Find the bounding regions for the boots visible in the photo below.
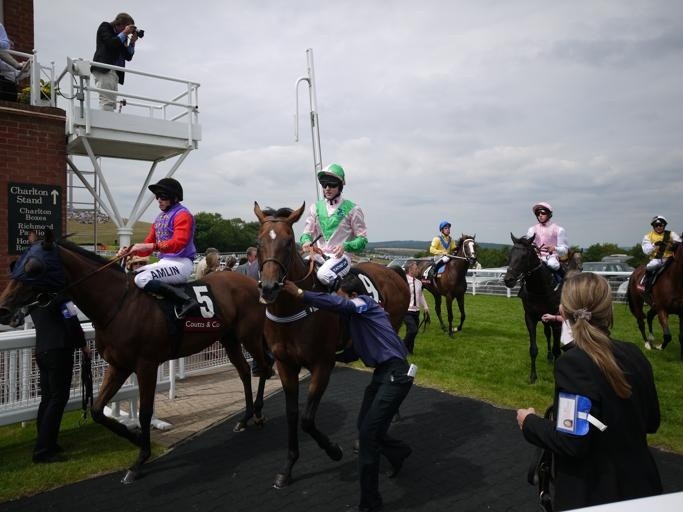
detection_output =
[144,280,199,321]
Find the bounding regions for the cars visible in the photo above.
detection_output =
[582,254,637,302]
[357,257,372,265]
[386,259,434,272]
[466,267,522,296]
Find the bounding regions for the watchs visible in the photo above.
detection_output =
[295,288,303,299]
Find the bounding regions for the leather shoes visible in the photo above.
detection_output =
[386,445,412,477]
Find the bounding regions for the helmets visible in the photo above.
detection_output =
[317,164,345,186]
[148,178,183,202]
[439,220,451,231]
[534,209,548,215]
[650,215,668,226]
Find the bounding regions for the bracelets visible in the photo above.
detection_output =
[555,316,557,322]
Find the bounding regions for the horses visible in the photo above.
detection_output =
[253,200,411,491]
[0,227,269,485]
[416,233,478,339]
[504,232,566,385]
[564,248,584,273]
[626,240,683,360]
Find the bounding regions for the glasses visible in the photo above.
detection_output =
[156,191,169,201]
[320,179,339,189]
[531,201,552,213]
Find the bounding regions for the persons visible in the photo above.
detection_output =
[29,299,92,465]
[90,13,138,111]
[0,21,30,102]
[116,177,200,318]
[301,163,368,292]
[526,202,569,279]
[637,215,682,291]
[403,260,430,354]
[516,272,664,511]
[282,273,414,511]
[126,246,261,284]
[427,221,457,285]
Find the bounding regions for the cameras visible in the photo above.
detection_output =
[133,28,144,39]
